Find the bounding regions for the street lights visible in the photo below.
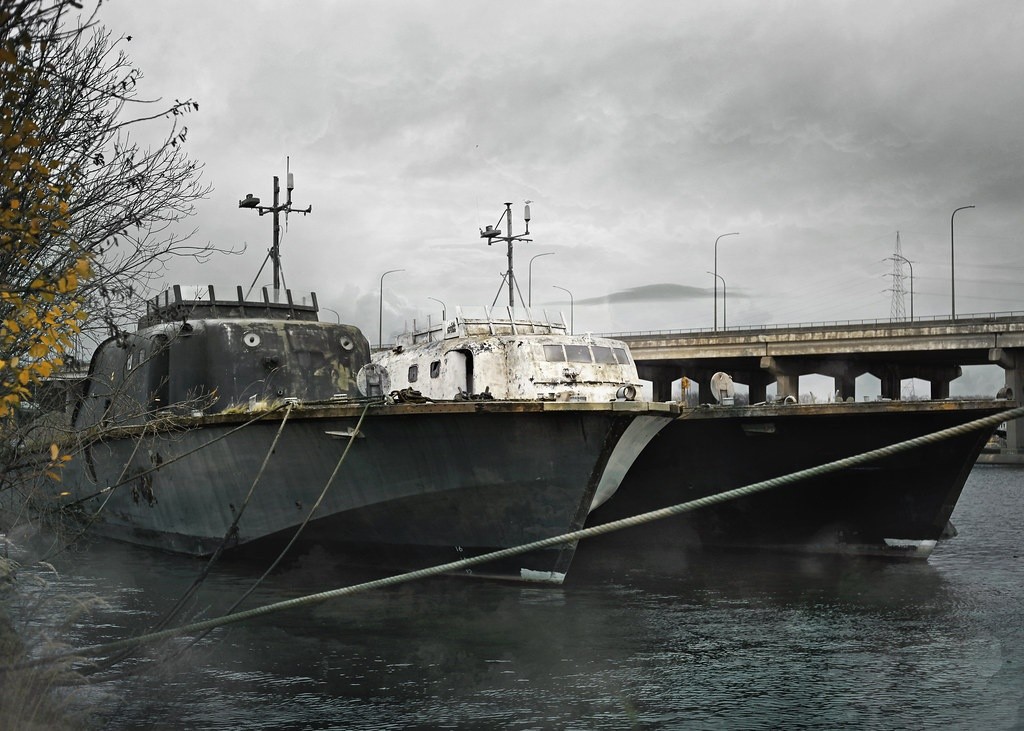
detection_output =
[707,272,726,331]
[428,297,446,321]
[553,286,573,335]
[893,254,913,322]
[715,233,739,331]
[951,205,975,320]
[380,269,406,349]
[529,253,555,307]
[322,308,339,324]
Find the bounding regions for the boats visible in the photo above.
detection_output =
[370,200,1018,562]
[0,156,684,584]
